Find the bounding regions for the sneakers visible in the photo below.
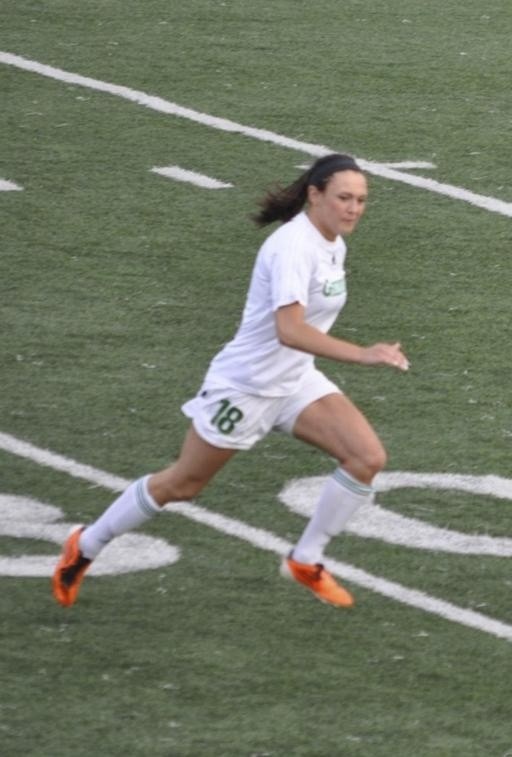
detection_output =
[53,524,94,609]
[279,547,356,609]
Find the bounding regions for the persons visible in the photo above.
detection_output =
[50,154,410,608]
